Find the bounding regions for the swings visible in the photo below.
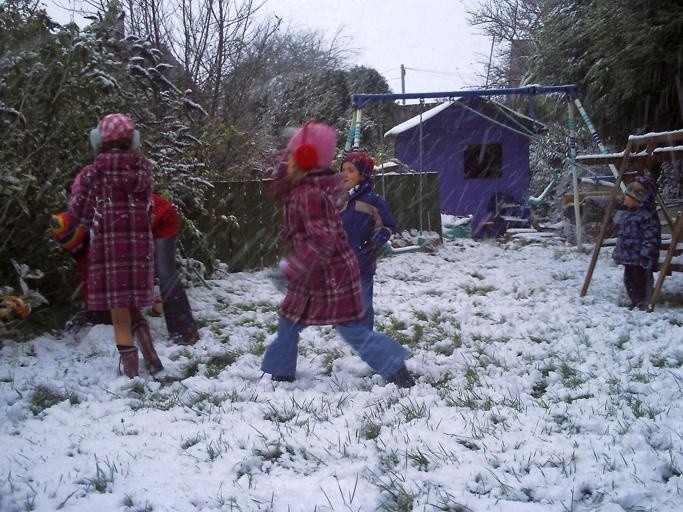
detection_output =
[484,94,536,225]
[453,95,616,188]
[378,98,425,254]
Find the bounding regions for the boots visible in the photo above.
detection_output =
[116,345,139,379]
[133,320,163,372]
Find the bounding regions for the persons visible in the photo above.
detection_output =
[261,122,415,387]
[58,113,163,378]
[50,188,199,345]
[612,177,659,308]
[335,153,395,329]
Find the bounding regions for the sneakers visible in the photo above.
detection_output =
[177,331,199,346]
[394,366,412,387]
[271,377,296,382]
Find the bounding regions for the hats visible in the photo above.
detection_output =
[288,125,337,169]
[624,181,647,203]
[97,114,135,149]
[51,213,88,255]
[340,150,374,181]
